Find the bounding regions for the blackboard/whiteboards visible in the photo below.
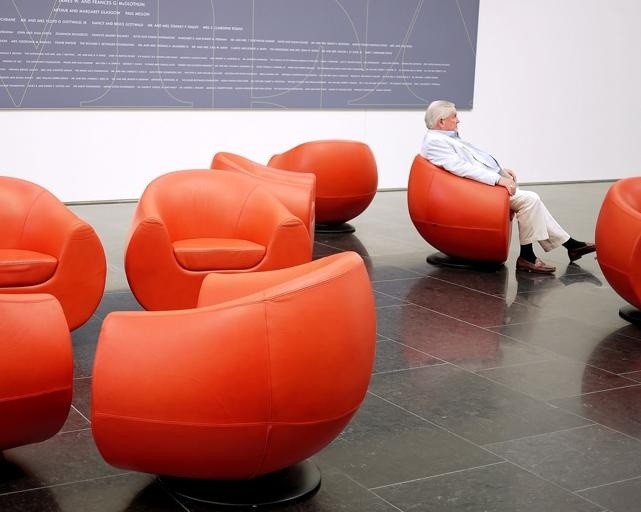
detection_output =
[0,0,480,111]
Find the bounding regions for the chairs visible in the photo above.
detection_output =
[594,177,641,328]
[270,142,378,236]
[210,152,317,252]
[0,176,108,331]
[91,253,377,512]
[125,170,316,311]
[408,154,513,271]
[0,293,73,452]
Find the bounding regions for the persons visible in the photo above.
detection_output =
[476,259,604,372]
[418,98,597,274]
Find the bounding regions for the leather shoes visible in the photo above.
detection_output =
[568,240,596,261]
[516,254,556,274]
